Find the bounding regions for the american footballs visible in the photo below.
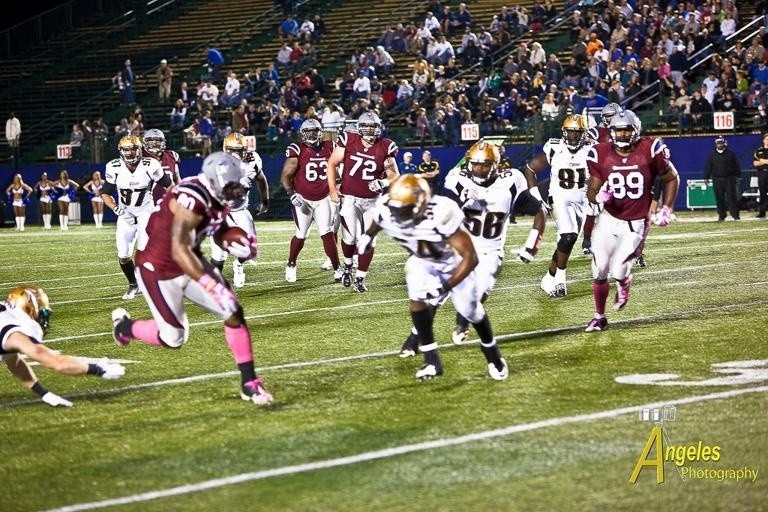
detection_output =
[214,227,248,251]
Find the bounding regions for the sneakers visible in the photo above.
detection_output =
[321,258,369,294]
[122,285,139,301]
[510,247,536,262]
[540,246,645,331]
[285,260,297,282]
[240,376,274,407]
[399,325,509,381]
[111,307,131,347]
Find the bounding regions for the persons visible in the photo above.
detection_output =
[110,151,274,408]
[398,141,548,361]
[356,170,510,381]
[0,0,767,300]
[585,111,680,334]
[1,284,127,407]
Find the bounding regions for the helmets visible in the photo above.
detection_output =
[561,103,642,152]
[117,128,166,165]
[465,142,501,185]
[383,174,431,229]
[343,111,383,145]
[201,132,256,212]
[300,119,323,150]
[5,285,52,336]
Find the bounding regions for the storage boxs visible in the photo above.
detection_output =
[686,178,719,210]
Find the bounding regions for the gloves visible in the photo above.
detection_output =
[329,201,341,233]
[85,357,126,380]
[197,273,239,313]
[368,178,390,192]
[222,234,258,263]
[650,205,673,227]
[457,188,478,209]
[286,187,305,207]
[352,235,373,269]
[29,379,74,407]
[528,185,552,217]
[418,282,455,308]
[594,180,615,212]
[111,204,127,218]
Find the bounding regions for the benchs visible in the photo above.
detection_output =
[742,176,767,205]
[1,0,768,161]
